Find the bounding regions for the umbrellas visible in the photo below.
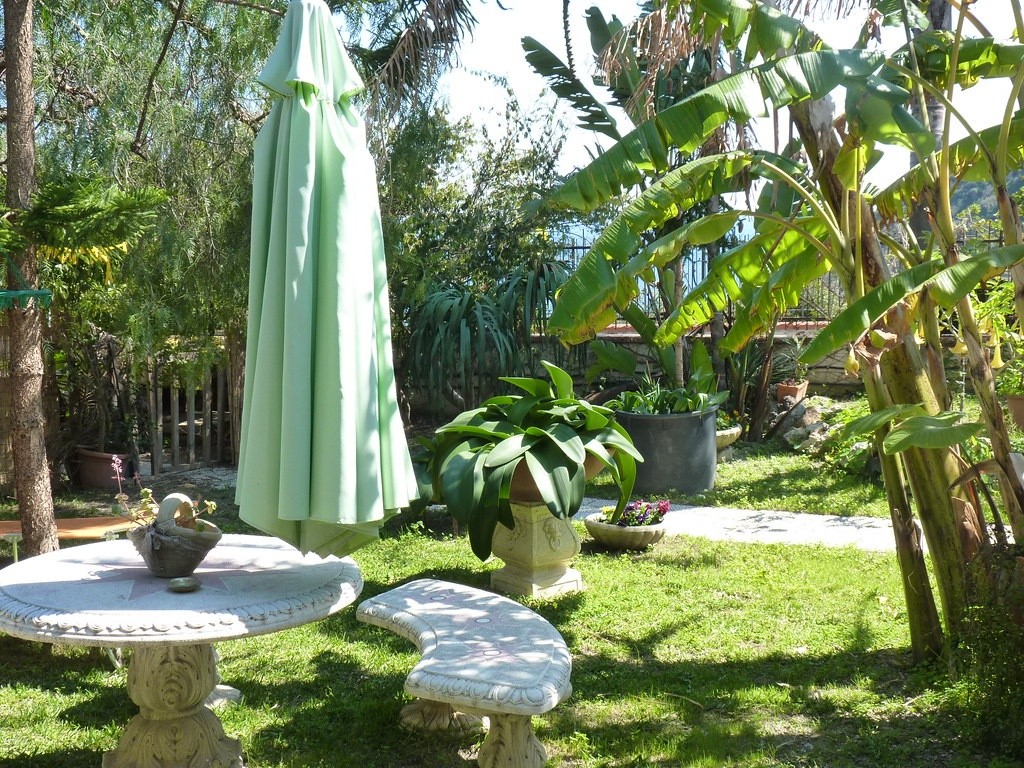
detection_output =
[235,0,425,559]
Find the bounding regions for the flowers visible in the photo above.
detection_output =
[600,489,680,527]
[102,455,218,541]
[716,408,741,431]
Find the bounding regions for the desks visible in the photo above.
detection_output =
[2,533,364,767]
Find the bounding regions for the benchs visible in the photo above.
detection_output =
[355,579,573,767]
[0,515,157,564]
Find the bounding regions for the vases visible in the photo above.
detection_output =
[585,511,666,548]
[716,423,742,450]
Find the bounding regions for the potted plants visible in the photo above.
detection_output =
[601,363,729,495]
[408,359,648,564]
[996,360,1024,432]
[776,334,809,403]
[58,324,151,489]
